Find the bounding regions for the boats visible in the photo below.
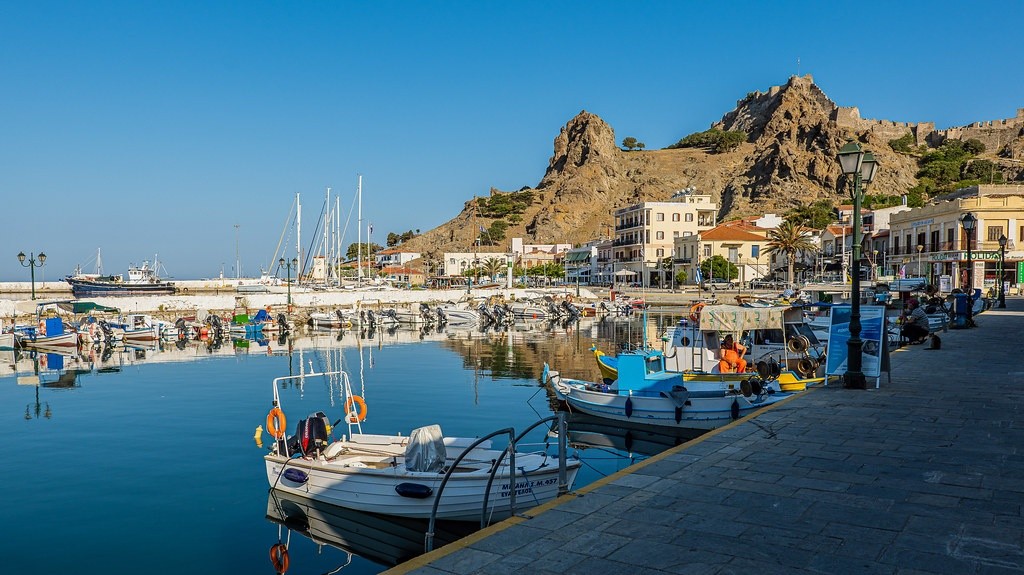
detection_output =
[63,247,178,297]
[541,359,796,430]
[702,278,735,291]
[748,276,797,290]
[544,407,712,458]
[267,369,581,525]
[1,286,646,371]
[661,284,989,378]
[266,488,484,568]
[590,310,840,397]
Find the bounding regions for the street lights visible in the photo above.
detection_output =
[17,250,46,300]
[873,250,879,265]
[233,224,242,280]
[917,245,924,279]
[997,234,1008,308]
[756,256,760,278]
[960,212,979,329]
[834,137,882,391]
[738,253,743,285]
[572,260,583,296]
[461,260,476,300]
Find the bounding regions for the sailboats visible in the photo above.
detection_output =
[255,174,374,291]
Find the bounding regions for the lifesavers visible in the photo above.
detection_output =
[343,394,368,423]
[266,408,287,438]
[688,302,708,321]
[270,543,289,573]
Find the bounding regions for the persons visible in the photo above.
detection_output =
[901,299,930,344]
[720,334,747,373]
